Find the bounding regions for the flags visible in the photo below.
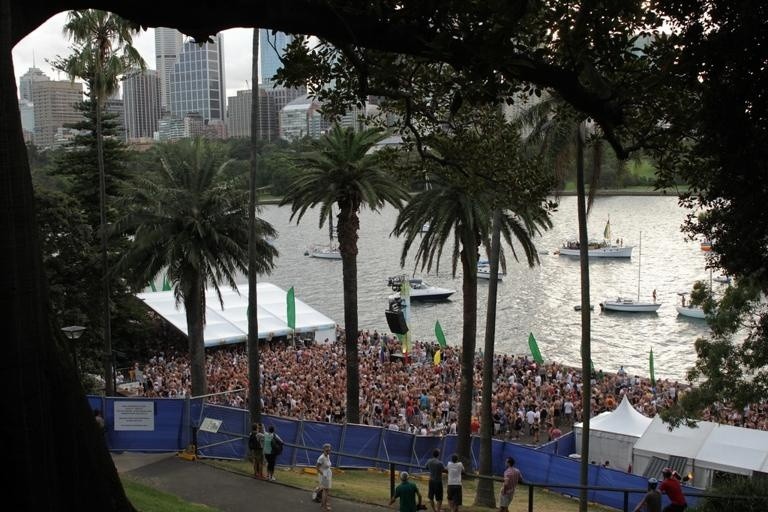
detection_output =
[604,221,611,239]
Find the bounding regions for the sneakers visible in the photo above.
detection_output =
[254,472,276,482]
[319,502,332,512]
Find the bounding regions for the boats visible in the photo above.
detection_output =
[557,212,638,261]
[381,272,456,304]
[474,258,504,282]
[418,221,431,233]
[303,226,341,263]
[712,276,730,283]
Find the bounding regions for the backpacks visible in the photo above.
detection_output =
[248,432,260,450]
[271,433,284,455]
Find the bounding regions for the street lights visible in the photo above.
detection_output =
[59,323,88,381]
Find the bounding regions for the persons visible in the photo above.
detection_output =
[633,478,661,512]
[447,455,465,512]
[498,457,522,512]
[656,468,687,512]
[563,237,622,249]
[389,472,422,512]
[425,450,448,512]
[653,289,656,302]
[317,444,333,511]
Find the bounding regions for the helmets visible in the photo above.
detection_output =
[662,468,672,474]
[648,477,658,484]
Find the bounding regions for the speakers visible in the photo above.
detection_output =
[385,310,408,335]
[391,353,412,365]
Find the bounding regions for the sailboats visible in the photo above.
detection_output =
[674,229,735,322]
[599,229,662,315]
